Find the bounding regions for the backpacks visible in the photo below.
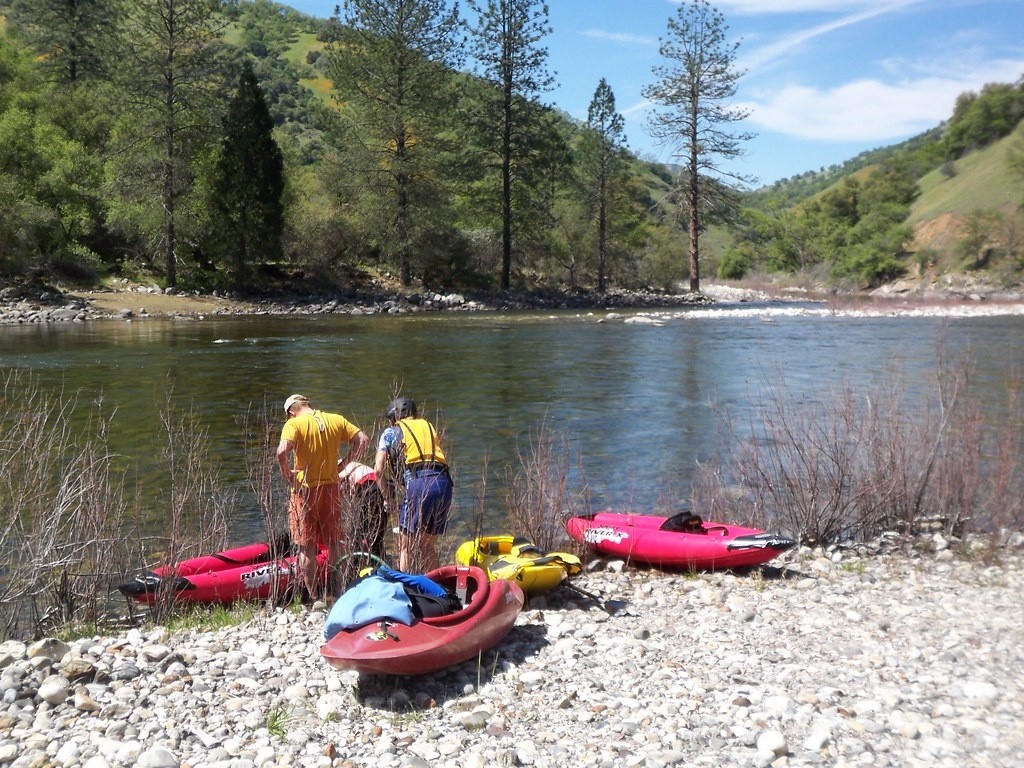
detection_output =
[374,566,462,617]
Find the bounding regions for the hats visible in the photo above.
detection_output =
[283,394,311,421]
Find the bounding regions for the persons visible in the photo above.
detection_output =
[338,459,390,572]
[374,397,452,576]
[277,394,370,605]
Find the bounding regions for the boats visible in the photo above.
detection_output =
[319,565,525,675]
[566,513,797,572]
[457,534,583,592]
[118,532,371,606]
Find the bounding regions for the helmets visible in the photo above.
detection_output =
[385,397,417,419]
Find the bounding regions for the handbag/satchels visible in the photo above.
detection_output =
[659,511,702,533]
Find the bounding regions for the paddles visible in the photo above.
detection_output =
[563,579,626,617]
